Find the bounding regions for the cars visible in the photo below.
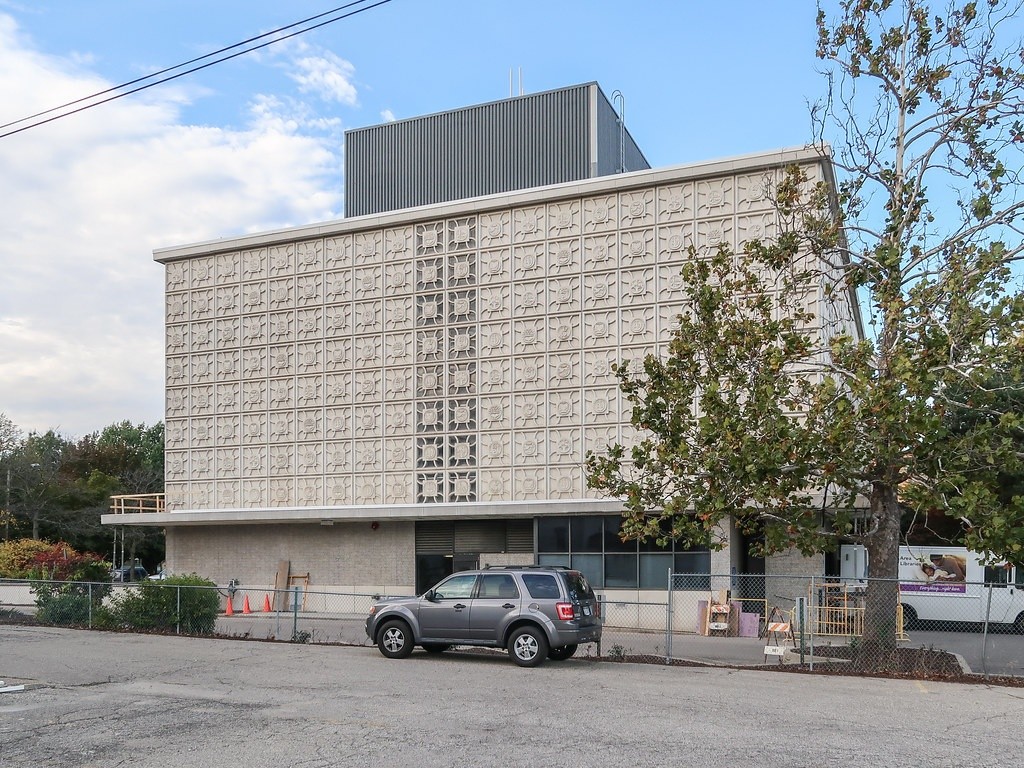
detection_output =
[110,564,148,583]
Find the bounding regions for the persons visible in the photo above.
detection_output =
[922,554,965,584]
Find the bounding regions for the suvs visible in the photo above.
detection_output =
[363,564,602,668]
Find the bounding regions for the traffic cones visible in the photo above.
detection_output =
[241,593,252,614]
[224,594,235,617]
[264,594,273,613]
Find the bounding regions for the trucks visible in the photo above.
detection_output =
[839,544,1023,631]
